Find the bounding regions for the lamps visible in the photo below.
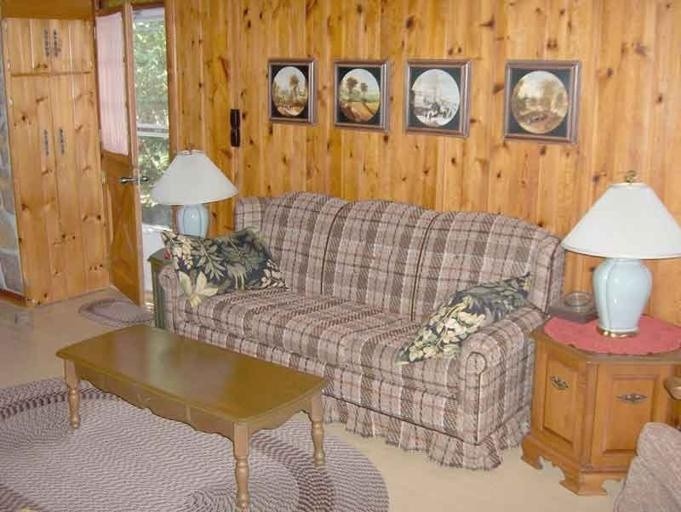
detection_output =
[561,171,681,340]
[148,143,237,242]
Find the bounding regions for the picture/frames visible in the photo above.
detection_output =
[331,59,389,132]
[502,58,581,144]
[402,57,472,138]
[266,58,316,126]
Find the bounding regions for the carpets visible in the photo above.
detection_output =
[0,375,391,511]
[78,295,154,332]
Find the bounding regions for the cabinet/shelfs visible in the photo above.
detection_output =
[0,0,112,308]
[146,245,179,333]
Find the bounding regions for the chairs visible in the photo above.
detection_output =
[610,420,680,512]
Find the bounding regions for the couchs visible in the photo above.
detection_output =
[144,190,564,472]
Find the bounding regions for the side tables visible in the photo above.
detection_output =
[518,314,681,496]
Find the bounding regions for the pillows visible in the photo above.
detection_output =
[157,225,288,308]
[398,269,531,367]
